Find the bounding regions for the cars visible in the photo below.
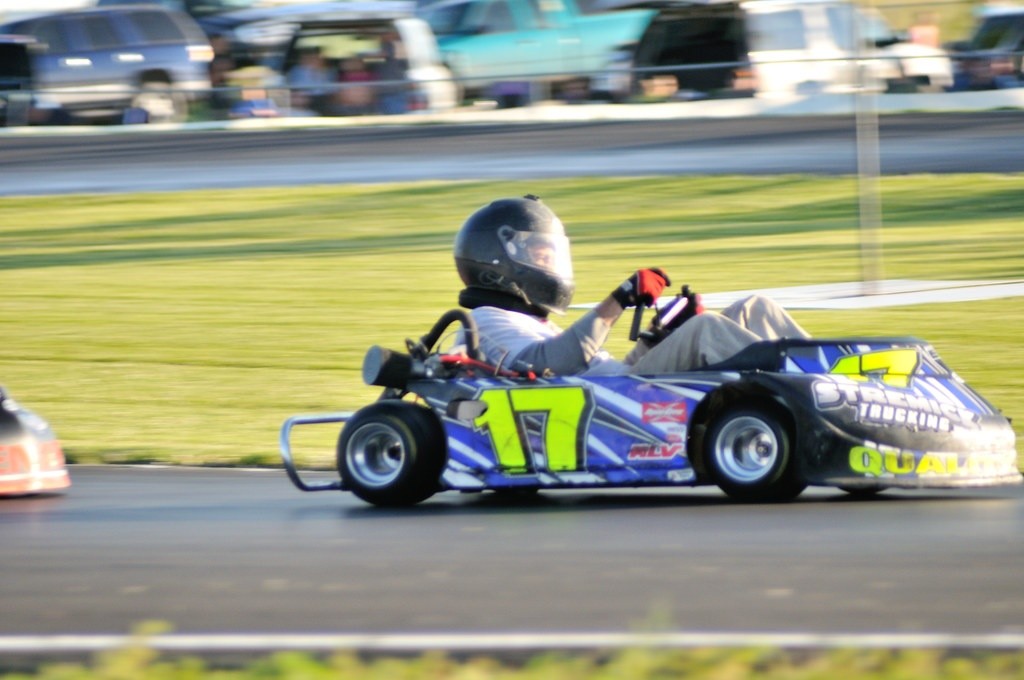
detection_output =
[0,0,1024,127]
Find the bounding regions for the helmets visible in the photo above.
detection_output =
[454,193,571,315]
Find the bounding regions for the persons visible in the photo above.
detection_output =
[122,29,380,124]
[454,192,814,379]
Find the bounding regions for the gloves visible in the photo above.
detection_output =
[649,285,704,336]
[612,267,669,309]
